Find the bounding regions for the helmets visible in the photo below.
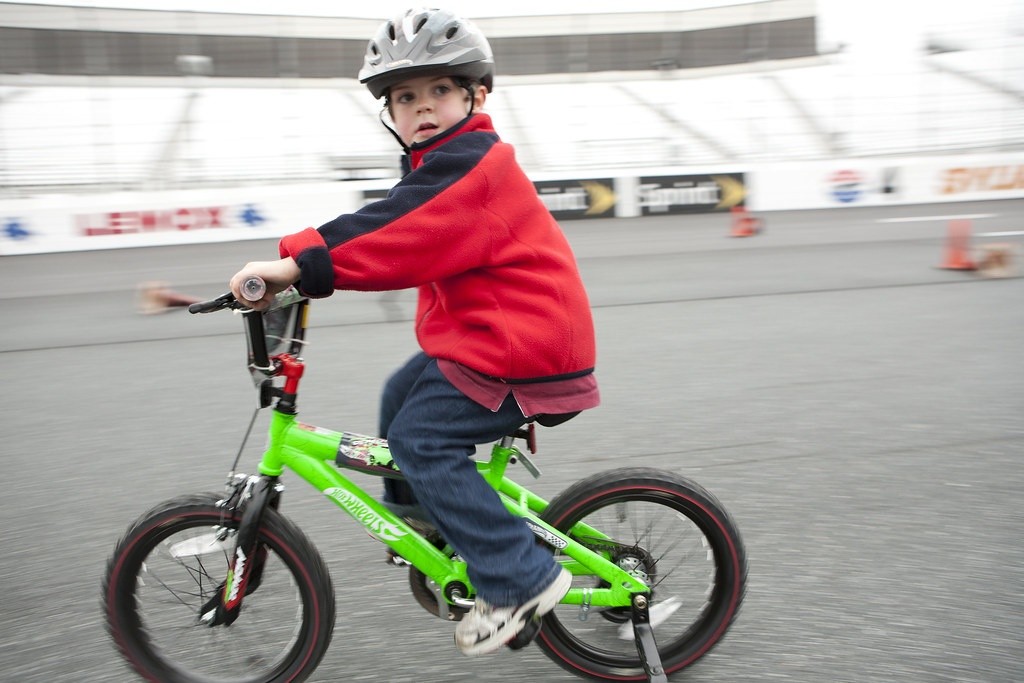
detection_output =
[359,7,494,101]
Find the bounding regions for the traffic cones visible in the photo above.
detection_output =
[726,200,758,238]
[934,216,978,271]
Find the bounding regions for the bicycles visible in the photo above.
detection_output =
[103,276,751,683]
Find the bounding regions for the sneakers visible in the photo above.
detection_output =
[455,568,572,657]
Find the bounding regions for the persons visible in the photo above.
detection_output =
[230,7,599,657]
